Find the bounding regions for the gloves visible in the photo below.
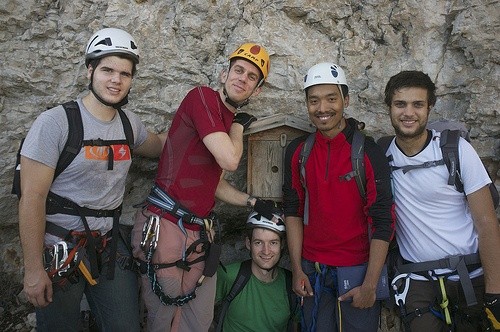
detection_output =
[481,291,500,332]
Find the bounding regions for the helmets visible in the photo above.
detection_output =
[246,211,285,236]
[302,63,348,91]
[229,43,270,79]
[86,28,140,64]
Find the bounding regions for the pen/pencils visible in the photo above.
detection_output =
[301,281,305,307]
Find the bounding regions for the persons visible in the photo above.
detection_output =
[284,63,395,332]
[132,43,271,332]
[214,211,304,332]
[13,27,169,331]
[373,70,500,332]
